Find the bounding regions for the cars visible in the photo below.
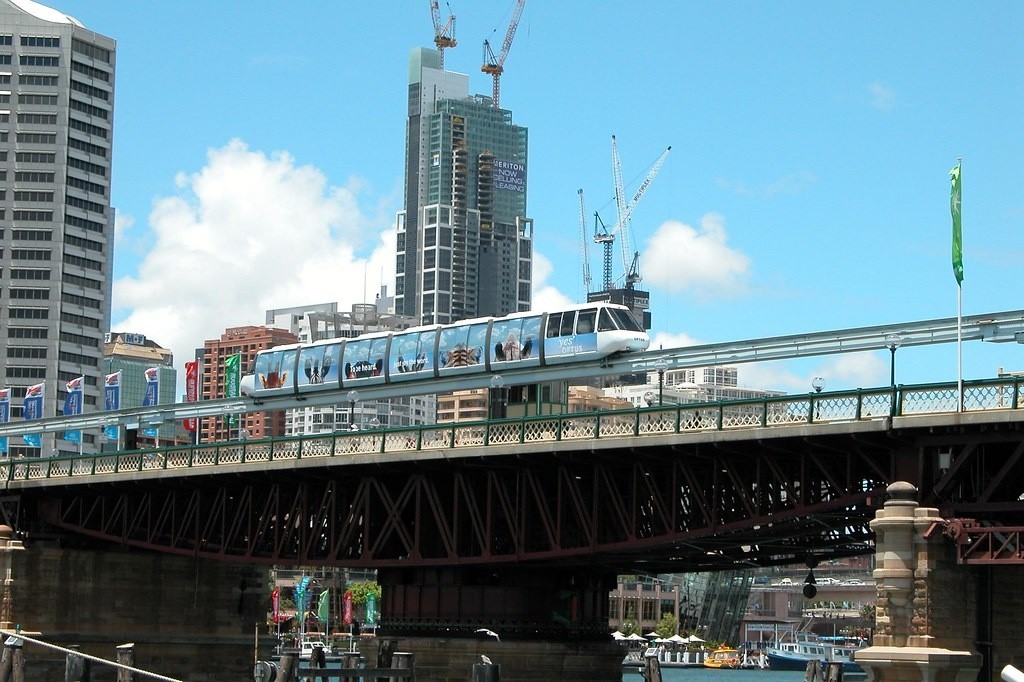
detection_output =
[732,576,866,586]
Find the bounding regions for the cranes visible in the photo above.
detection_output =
[479,0,529,107]
[575,132,672,297]
[428,0,458,69]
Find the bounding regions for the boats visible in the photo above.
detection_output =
[703,649,741,668]
[766,641,864,672]
[298,642,333,657]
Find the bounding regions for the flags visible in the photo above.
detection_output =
[0,388,11,452]
[365,593,376,625]
[142,367,159,436]
[23,383,44,447]
[63,377,83,444]
[343,590,352,624]
[318,590,329,622]
[183,361,198,431]
[272,589,279,622]
[104,371,120,440]
[224,354,239,426]
[949,162,964,288]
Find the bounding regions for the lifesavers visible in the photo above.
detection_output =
[333,646,337,649]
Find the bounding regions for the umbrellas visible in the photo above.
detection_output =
[611,631,706,649]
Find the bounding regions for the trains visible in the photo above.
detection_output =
[239,301,651,398]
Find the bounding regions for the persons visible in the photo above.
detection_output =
[0,448,60,478]
[293,635,299,647]
[502,333,523,361]
